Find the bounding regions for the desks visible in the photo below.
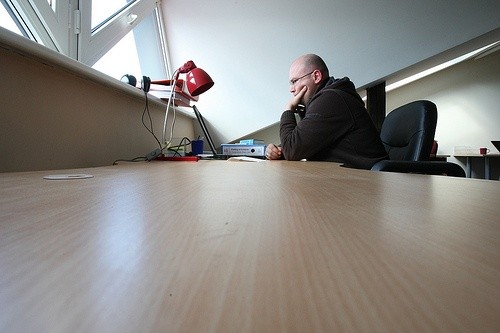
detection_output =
[0,155,500,333]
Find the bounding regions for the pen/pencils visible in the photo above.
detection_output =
[197,135,201,140]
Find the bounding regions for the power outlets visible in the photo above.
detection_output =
[162,137,185,151]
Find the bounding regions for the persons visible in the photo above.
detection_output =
[265,53,389,169]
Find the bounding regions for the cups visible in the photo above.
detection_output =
[479,148,490,156]
[190,139,204,156]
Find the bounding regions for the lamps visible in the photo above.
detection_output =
[154,60,214,162]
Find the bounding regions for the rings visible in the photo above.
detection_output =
[265,153,268,159]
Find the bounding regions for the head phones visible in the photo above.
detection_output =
[120,74,151,92]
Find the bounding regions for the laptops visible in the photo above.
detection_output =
[491,141,500,153]
[192,105,267,160]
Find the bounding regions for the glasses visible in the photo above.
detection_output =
[288,70,314,85]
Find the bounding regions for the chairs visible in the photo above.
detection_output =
[370,100,466,178]
